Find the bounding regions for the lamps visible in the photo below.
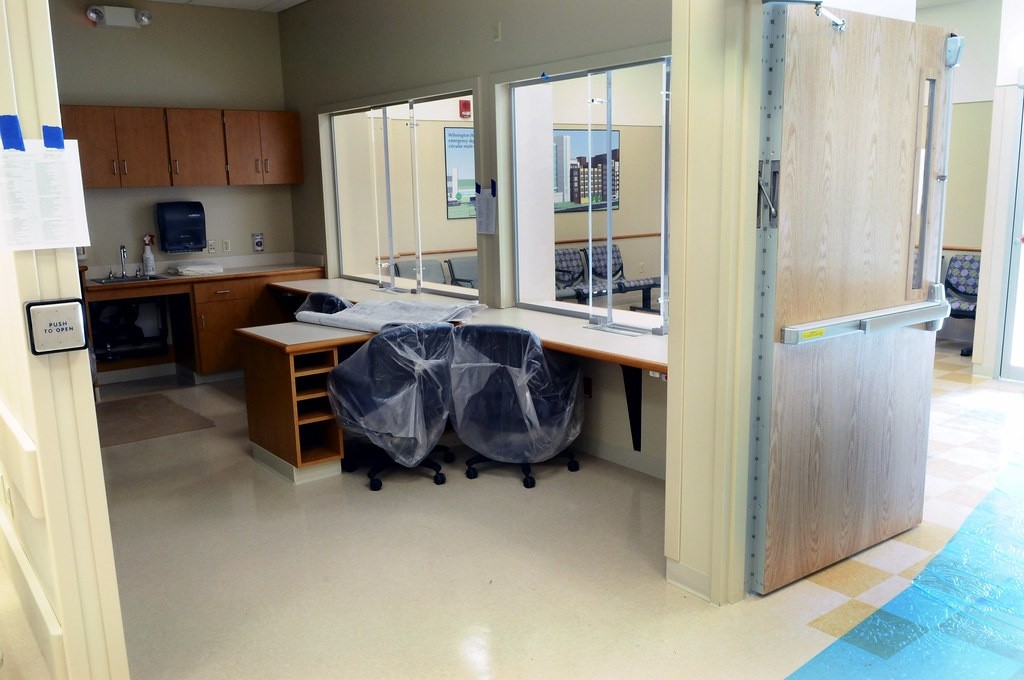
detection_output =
[88,5,152,31]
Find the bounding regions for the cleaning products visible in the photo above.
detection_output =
[143,234,155,275]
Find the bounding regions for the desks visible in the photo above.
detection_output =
[234,320,378,468]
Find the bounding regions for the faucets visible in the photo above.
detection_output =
[119,245,128,279]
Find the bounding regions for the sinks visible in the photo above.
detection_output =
[90,275,168,285]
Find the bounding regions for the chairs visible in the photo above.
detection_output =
[328,321,455,491]
[451,324,581,488]
[944,253,981,356]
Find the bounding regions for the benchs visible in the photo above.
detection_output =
[393,243,661,313]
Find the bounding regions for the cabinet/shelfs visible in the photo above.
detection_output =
[61,105,173,187]
[222,109,304,186]
[166,107,228,186]
[192,268,324,377]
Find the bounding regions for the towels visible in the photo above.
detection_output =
[168,262,224,276]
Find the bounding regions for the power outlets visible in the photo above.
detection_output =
[209,241,216,253]
[222,239,231,252]
